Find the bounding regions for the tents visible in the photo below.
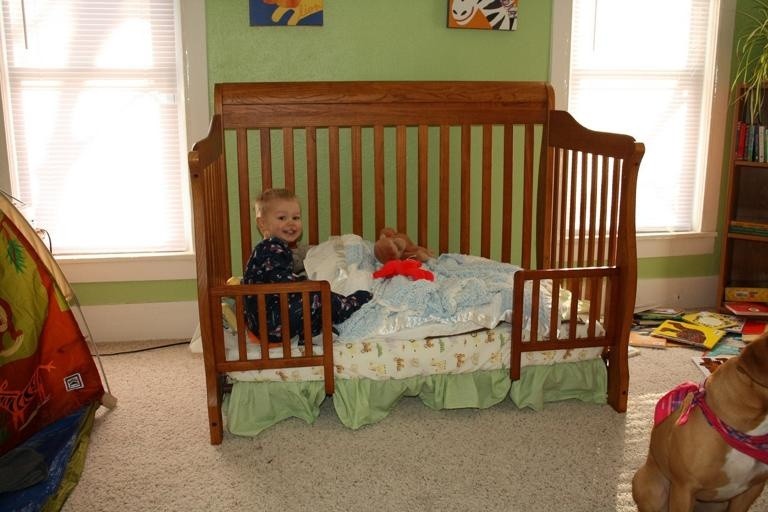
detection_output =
[0,191,117,512]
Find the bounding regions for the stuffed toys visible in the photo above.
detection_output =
[376,227,433,262]
[373,258,434,283]
[292,243,317,274]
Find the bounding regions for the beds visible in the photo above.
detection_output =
[187,81,645,446]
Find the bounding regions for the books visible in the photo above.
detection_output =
[742,320,768,341]
[743,126,749,161]
[730,217,768,230]
[735,121,742,160]
[637,319,664,325]
[730,231,768,237]
[765,128,768,163]
[633,305,656,314]
[628,332,667,348]
[738,123,746,160]
[651,319,727,350]
[691,355,738,376]
[698,312,747,333]
[759,125,767,163]
[638,307,682,320]
[748,125,754,161]
[702,334,749,356]
[627,346,639,358]
[755,125,759,161]
[666,339,696,347]
[729,226,768,234]
[724,287,768,302]
[724,301,768,315]
[700,314,738,329]
[682,312,699,324]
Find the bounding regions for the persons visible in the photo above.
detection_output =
[241,188,372,347]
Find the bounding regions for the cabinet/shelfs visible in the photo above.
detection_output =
[715,81,768,319]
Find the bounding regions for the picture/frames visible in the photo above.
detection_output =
[447,0,519,32]
[249,0,325,29]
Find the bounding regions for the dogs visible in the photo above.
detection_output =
[632,329,768,512]
